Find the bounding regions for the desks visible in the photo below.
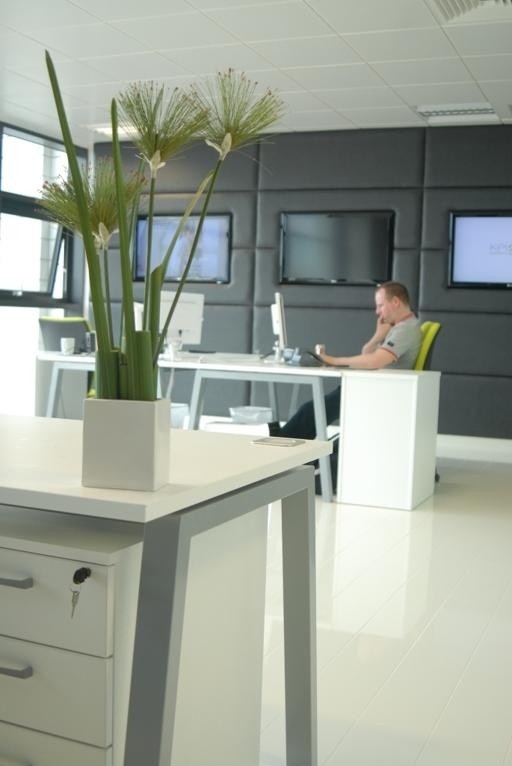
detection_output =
[0,413,333,766]
[35,350,441,511]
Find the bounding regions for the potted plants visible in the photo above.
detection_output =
[31,49,290,494]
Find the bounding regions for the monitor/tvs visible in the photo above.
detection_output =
[160,290,205,363]
[279,209,395,287]
[264,292,288,362]
[133,213,231,283]
[448,210,512,290]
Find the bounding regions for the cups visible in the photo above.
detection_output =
[60,337,75,355]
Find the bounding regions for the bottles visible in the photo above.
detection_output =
[84,332,95,353]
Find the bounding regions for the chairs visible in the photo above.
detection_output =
[411,320,442,483]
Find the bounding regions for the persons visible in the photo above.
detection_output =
[276,279,425,441]
